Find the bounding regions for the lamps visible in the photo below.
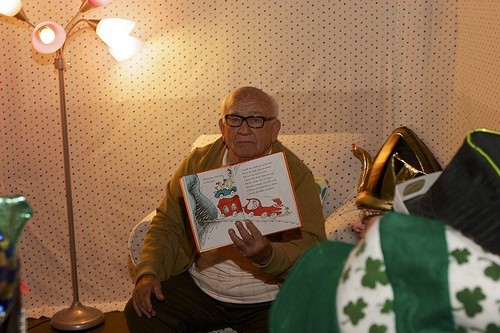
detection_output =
[0,0,143,331]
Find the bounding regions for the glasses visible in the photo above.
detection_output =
[225,114,274,128]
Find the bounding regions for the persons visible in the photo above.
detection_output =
[122,85,326,333]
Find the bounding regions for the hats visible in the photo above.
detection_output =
[351,127,443,210]
[389,129,500,259]
[267,213,499,333]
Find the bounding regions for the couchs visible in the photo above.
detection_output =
[130,134,364,268]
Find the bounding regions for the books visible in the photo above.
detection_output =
[179,151,304,254]
